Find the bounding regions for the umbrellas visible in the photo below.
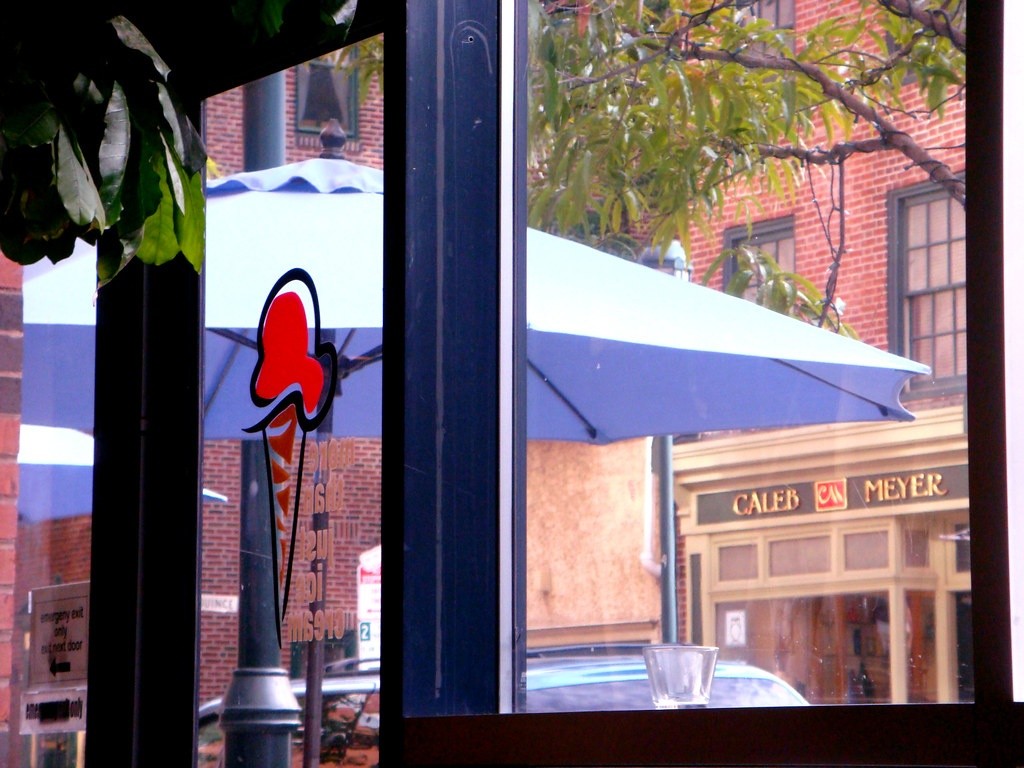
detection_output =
[17,421,228,523]
[20,119,932,768]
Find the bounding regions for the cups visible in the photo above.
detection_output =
[642,645,719,710]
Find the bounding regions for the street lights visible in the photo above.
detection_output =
[643,239,695,636]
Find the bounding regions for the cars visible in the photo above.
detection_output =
[527,640,809,713]
[195,656,381,768]
